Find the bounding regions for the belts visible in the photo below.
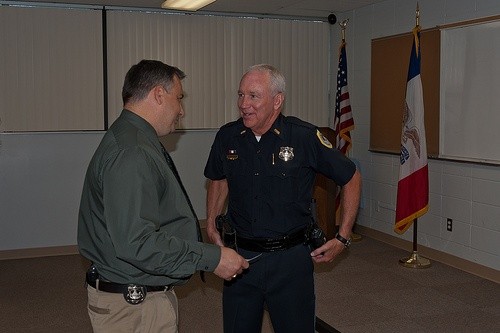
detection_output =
[85,272,175,294]
[221,226,307,253]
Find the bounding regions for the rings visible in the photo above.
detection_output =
[232,274,236,278]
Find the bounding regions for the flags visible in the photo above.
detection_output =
[334,43,354,225]
[394,26,430,233]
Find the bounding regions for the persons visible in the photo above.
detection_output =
[203,64,361,333]
[77,60,249,333]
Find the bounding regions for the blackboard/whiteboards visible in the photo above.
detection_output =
[434,19,500,164]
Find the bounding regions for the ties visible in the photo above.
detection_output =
[158,140,206,284]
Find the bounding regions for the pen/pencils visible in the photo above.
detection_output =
[272,152,274,164]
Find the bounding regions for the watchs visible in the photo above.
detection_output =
[335,233,352,248]
